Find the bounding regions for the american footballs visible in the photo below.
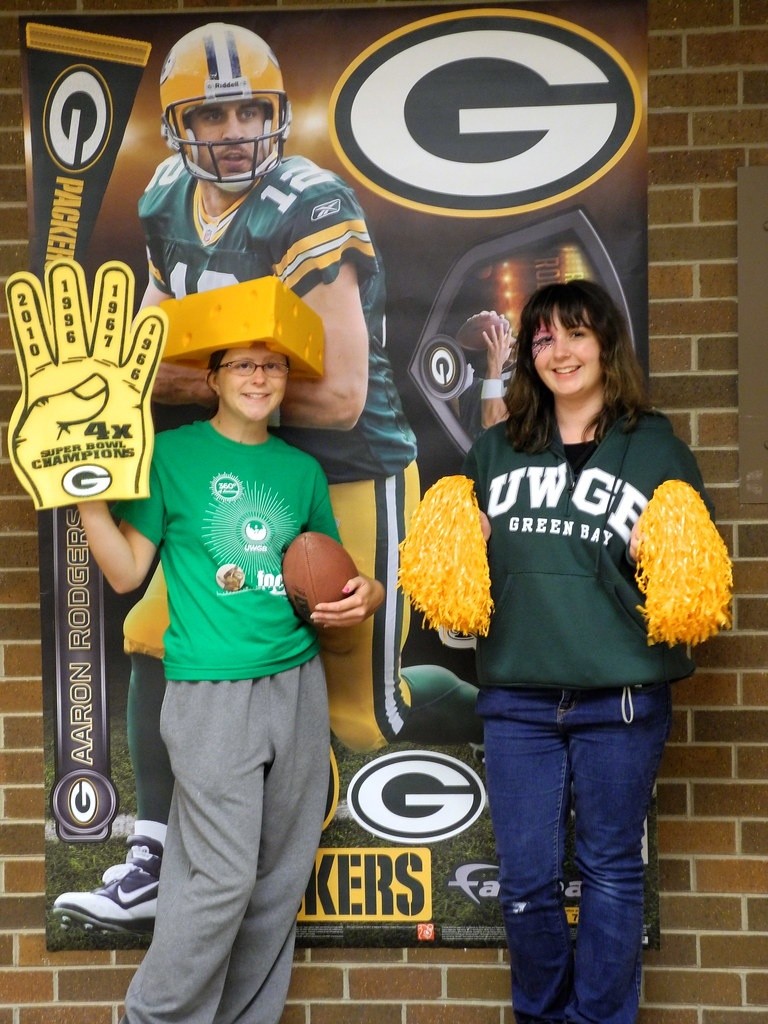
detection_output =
[282,532,359,633]
[456,314,508,357]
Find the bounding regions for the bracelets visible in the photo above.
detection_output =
[481,378,504,398]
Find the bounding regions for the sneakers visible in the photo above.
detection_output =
[462,742,486,778]
[53,835,163,936]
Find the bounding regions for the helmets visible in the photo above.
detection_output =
[159,23,293,192]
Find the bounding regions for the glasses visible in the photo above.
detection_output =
[216,360,290,377]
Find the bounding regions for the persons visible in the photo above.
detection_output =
[77,342,387,1024]
[463,281,713,1024]
[51,22,514,947]
